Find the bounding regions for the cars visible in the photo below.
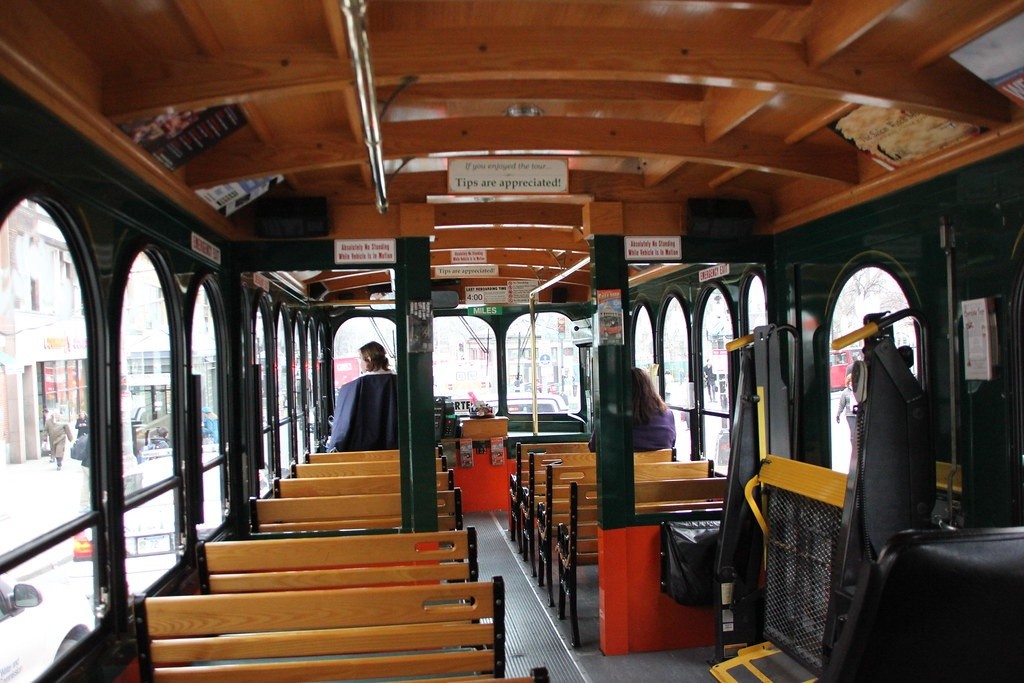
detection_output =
[72,451,222,574]
[0,573,96,683]
[486,392,570,416]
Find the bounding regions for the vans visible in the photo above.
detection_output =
[829,349,851,390]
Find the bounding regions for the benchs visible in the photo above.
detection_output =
[130,442,726,683]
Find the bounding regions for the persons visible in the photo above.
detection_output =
[353,341,394,379]
[75,413,88,439]
[588,367,676,453]
[836,373,859,444]
[151,427,170,448]
[835,354,844,364]
[704,359,718,403]
[42,410,73,471]
[665,370,674,402]
[201,406,218,444]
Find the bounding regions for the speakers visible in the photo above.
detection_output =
[686,197,755,239]
[309,283,329,301]
[252,196,330,239]
[551,288,567,304]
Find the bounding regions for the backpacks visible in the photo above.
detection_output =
[69,433,88,461]
